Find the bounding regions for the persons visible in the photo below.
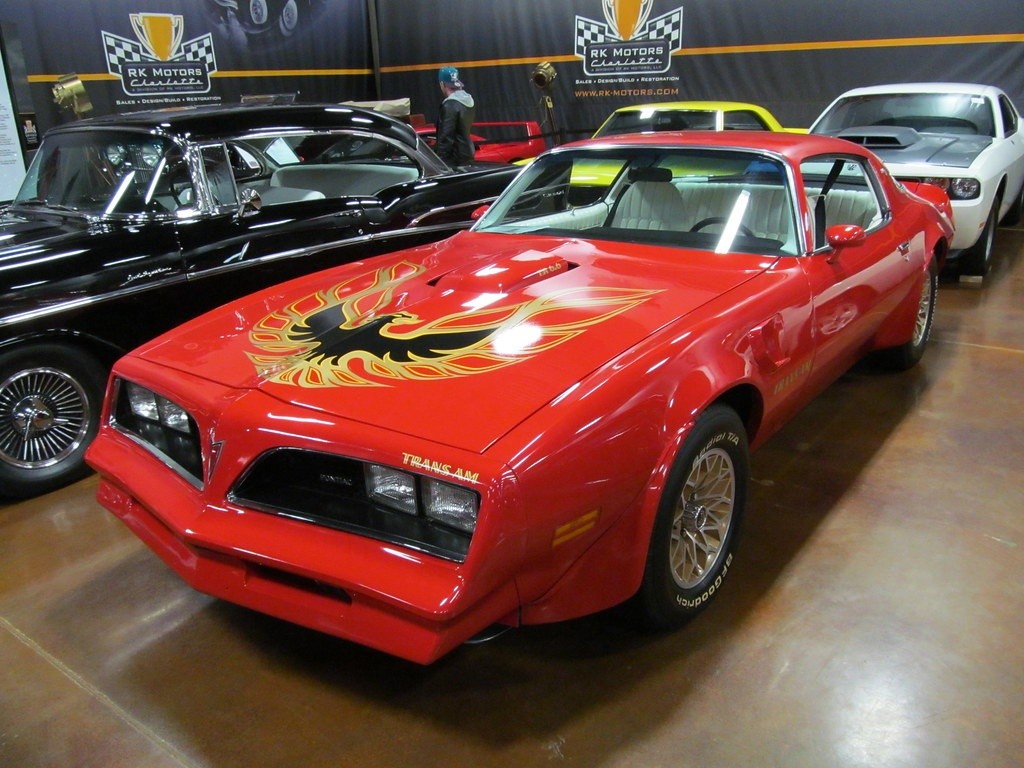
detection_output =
[435,67,476,161]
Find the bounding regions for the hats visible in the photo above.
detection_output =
[439,66,465,88]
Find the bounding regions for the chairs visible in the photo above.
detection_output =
[174,184,326,211]
[609,180,689,232]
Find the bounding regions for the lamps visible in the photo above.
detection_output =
[530,60,561,146]
[51,72,93,119]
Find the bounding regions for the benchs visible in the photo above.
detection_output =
[672,182,876,245]
[269,163,420,196]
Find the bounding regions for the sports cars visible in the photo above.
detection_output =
[516,100,786,208]
[89,130,956,669]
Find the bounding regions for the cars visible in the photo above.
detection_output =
[1,104,527,493]
[415,121,546,166]
[799,83,1024,275]
